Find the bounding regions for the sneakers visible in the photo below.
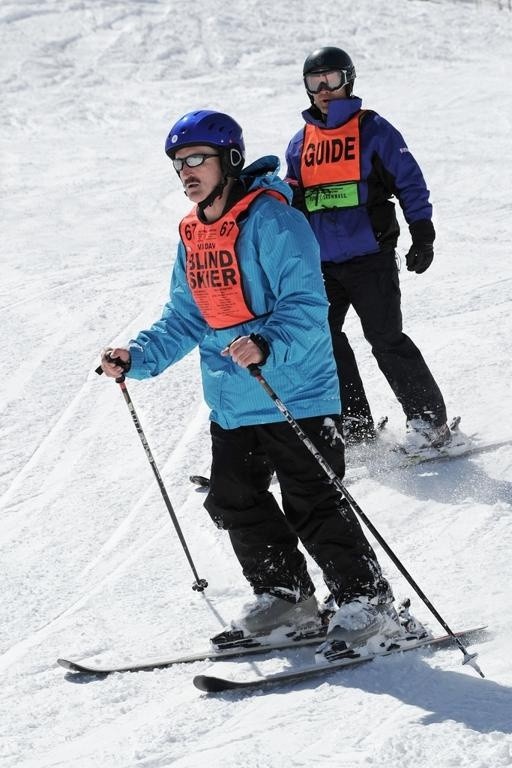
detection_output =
[400,424,450,455]
[231,594,318,636]
[327,600,399,641]
[341,418,373,445]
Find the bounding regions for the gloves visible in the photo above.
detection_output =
[406,218,435,273]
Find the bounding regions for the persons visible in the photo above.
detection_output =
[102,109,394,637]
[278,45,454,456]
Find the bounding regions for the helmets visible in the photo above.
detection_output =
[165,110,245,178]
[304,47,355,100]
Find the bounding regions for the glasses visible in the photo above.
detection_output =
[172,154,218,171]
[304,69,349,95]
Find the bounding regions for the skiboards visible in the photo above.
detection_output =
[189,427,510,494]
[58,605,490,693]
